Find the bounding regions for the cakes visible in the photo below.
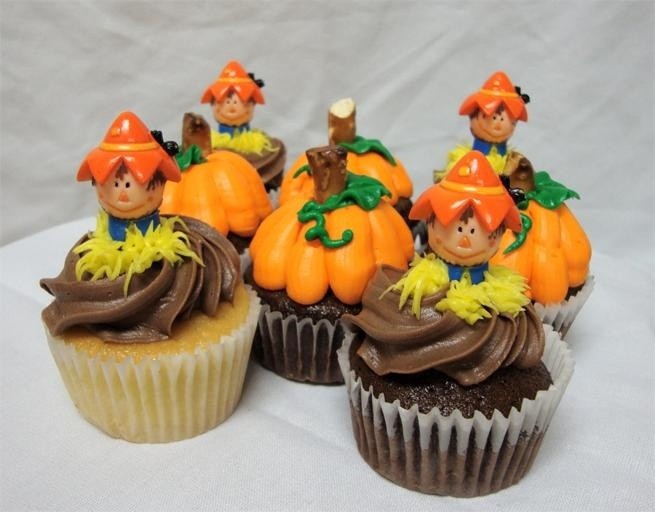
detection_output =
[275,95,413,224]
[341,150,563,494]
[451,70,598,340]
[193,62,282,192]
[235,141,419,387]
[31,111,261,444]
[151,112,273,265]
